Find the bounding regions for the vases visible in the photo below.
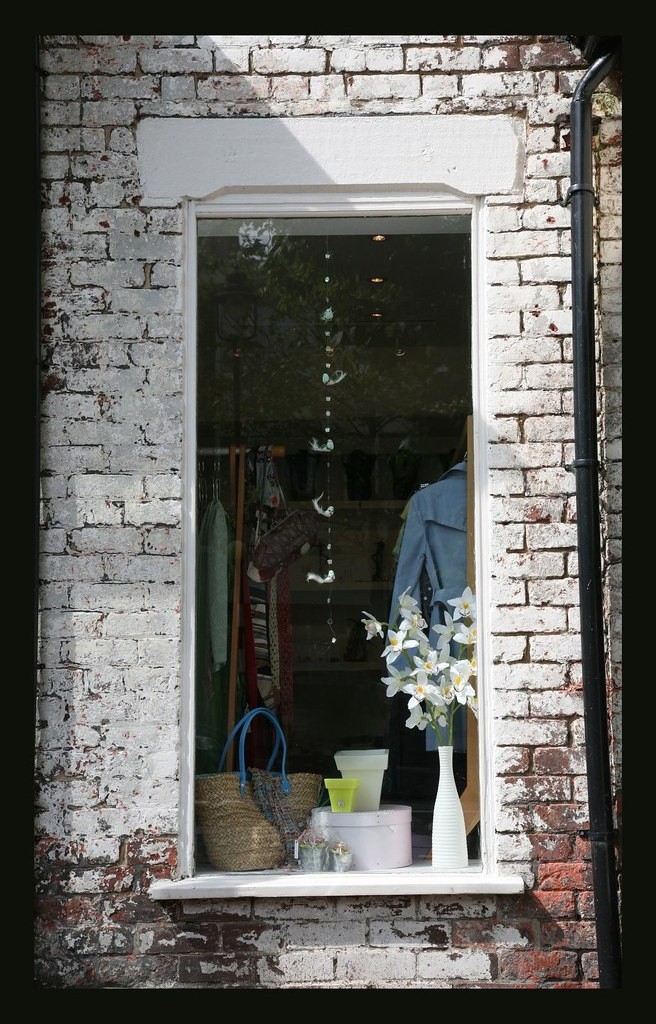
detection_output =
[431,746,469,869]
[334,749,389,810]
[325,778,362,813]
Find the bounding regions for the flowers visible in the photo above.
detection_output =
[360,586,480,746]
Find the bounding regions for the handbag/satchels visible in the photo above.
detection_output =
[246,446,318,585]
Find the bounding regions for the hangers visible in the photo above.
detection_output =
[196,446,224,514]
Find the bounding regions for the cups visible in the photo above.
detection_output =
[324,778,361,813]
[334,749,390,810]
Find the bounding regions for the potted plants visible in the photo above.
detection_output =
[329,840,353,872]
[300,838,329,871]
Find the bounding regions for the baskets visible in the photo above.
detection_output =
[196,708,321,871]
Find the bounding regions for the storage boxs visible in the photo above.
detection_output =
[310,804,413,871]
